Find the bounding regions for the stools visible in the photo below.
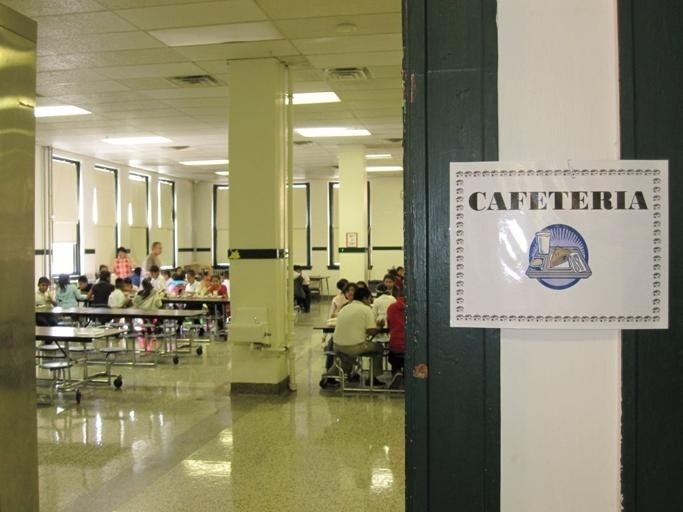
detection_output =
[35,321,208,410]
[323,349,406,394]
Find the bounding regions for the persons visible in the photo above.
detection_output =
[112,246,133,278]
[34,259,311,351]
[317,263,404,391]
[141,241,162,270]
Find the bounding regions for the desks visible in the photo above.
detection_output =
[312,323,390,389]
[35,296,234,368]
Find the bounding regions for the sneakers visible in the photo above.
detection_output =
[388,368,402,389]
[325,378,340,388]
[363,376,385,388]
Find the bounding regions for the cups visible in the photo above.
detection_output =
[213,290,217,296]
